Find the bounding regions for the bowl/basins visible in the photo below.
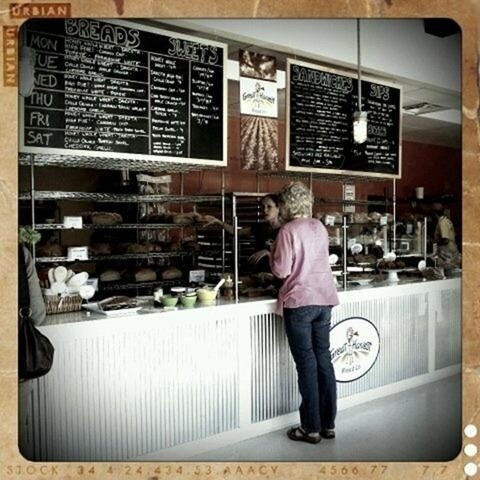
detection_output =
[159,289,218,308]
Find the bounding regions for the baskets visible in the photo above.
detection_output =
[41,288,83,314]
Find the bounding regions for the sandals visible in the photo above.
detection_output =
[287,426,335,444]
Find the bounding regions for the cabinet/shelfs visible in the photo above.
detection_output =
[19,157,435,296]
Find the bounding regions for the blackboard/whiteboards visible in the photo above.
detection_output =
[18,17,229,168]
[285,54,403,180]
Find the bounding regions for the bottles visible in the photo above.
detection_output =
[415,185,425,199]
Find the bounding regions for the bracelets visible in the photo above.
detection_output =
[221,222,227,229]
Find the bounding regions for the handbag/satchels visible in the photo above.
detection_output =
[18,316,54,379]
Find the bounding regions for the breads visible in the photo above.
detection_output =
[38,212,194,282]
[315,211,407,270]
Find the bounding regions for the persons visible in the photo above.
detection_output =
[429,201,460,270]
[19,242,48,442]
[268,180,340,445]
[194,191,284,288]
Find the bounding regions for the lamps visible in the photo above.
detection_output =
[346,18,369,145]
